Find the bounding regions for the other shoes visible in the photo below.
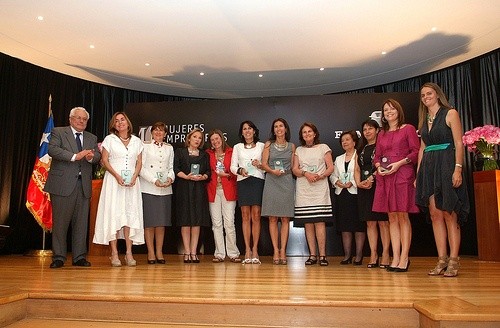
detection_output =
[191,253,200,263]
[156,259,165,264]
[212,258,224,263]
[110,254,122,267]
[124,254,137,266]
[279,259,287,265]
[340,256,352,264]
[72,258,91,266]
[319,255,328,266]
[273,259,279,265]
[305,255,317,265]
[147,259,156,264]
[354,257,363,265]
[184,254,192,263]
[241,258,252,264]
[252,258,261,264]
[49,260,63,268]
[230,258,241,263]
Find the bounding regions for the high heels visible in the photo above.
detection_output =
[379,264,389,269]
[443,257,461,277]
[428,255,449,276]
[386,267,395,272]
[367,254,380,268]
[394,258,411,272]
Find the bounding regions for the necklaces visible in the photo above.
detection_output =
[427,114,434,124]
[120,137,129,141]
[246,141,253,148]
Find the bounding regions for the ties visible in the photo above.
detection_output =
[75,133,82,153]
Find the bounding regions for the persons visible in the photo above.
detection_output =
[413,82,470,277]
[203,128,241,263]
[372,99,421,272]
[44,107,101,269]
[93,112,145,266]
[353,120,391,269]
[261,118,296,264]
[229,121,266,263]
[292,122,334,266]
[138,122,176,264]
[171,129,210,263]
[328,131,367,264]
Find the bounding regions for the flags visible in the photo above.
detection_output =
[25,116,54,234]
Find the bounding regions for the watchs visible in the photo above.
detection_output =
[405,158,410,163]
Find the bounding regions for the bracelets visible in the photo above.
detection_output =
[302,171,306,176]
[367,178,375,183]
[376,166,379,169]
[456,164,462,167]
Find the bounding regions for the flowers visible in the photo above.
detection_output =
[462,125,500,168]
[98,142,101,151]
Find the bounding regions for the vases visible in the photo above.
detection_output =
[94,164,104,179]
[475,148,499,171]
